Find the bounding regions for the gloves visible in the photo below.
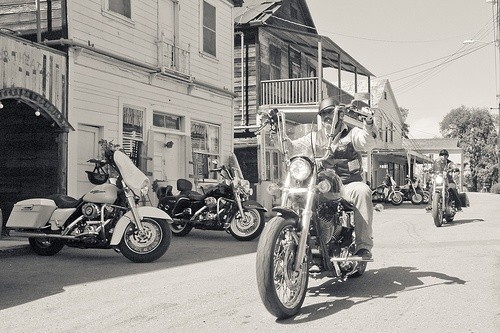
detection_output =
[267,109,281,134]
[358,106,374,124]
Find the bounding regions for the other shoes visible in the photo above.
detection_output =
[426,205,432,210]
[457,207,462,210]
[357,249,372,259]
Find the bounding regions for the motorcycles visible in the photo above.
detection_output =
[368,172,432,206]
[4,138,173,263]
[157,151,269,241]
[423,165,463,228]
[255,92,374,318]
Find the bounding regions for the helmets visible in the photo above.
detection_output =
[439,149,448,156]
[85,171,109,185]
[319,98,339,115]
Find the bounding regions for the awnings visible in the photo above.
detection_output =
[378,147,431,186]
[263,23,377,109]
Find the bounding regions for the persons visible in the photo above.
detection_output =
[258,97,377,258]
[470,165,477,192]
[423,150,462,210]
